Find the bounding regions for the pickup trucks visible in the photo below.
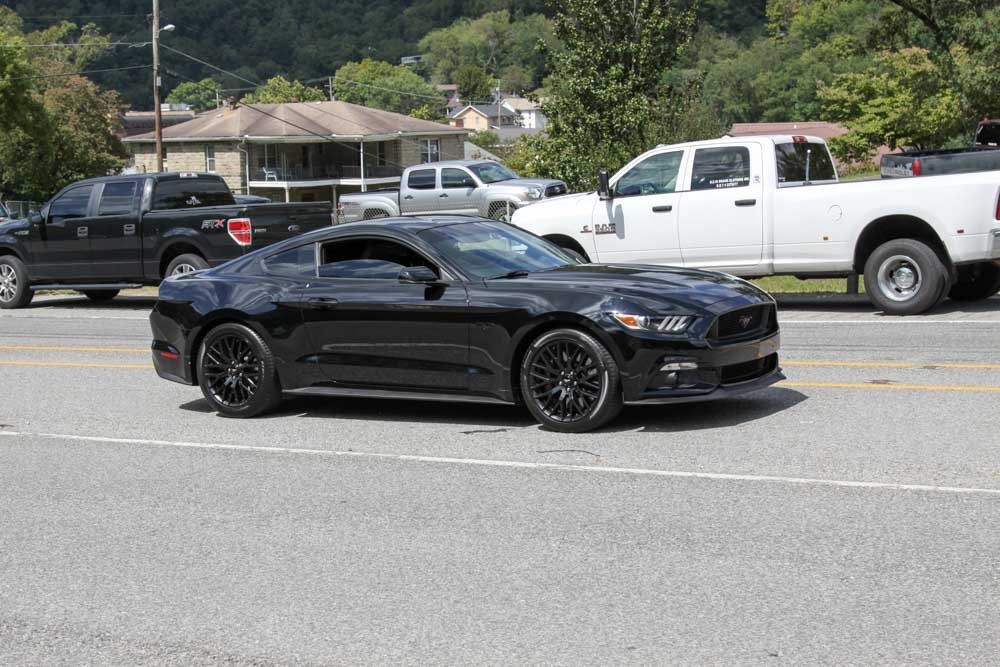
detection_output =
[879,118,1000,179]
[508,134,1000,314]
[336,159,569,226]
[0,168,334,310]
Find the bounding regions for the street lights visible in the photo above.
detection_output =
[152,23,178,173]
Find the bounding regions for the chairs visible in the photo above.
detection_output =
[263,167,277,181]
[291,163,303,180]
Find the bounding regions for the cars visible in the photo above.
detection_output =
[148,214,787,434]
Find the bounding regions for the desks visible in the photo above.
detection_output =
[273,168,294,181]
